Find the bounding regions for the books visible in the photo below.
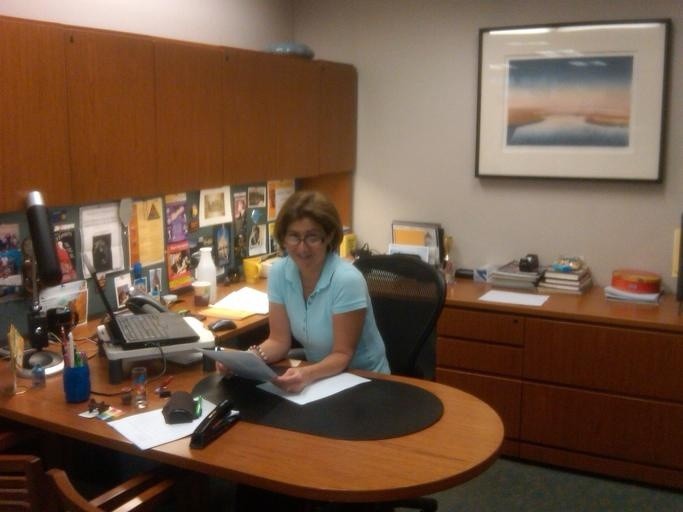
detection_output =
[454,259,667,307]
[386,218,456,287]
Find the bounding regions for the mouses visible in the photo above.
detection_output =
[208,319,237,332]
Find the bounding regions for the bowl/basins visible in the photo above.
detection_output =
[610,269,661,293]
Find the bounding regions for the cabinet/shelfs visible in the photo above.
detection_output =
[350,240,682,492]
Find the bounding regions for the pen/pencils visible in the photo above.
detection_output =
[154,375,174,394]
[61,325,87,369]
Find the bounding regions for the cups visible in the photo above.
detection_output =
[151,285,160,303]
[242,257,280,284]
[64,363,90,403]
[190,281,212,307]
[132,367,149,409]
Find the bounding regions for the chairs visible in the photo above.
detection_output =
[1,451,183,512]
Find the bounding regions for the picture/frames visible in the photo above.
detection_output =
[473,16,672,184]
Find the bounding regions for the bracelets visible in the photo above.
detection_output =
[246,344,270,367]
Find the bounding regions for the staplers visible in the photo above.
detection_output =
[190,399,241,448]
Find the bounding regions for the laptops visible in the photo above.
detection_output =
[83,254,200,351]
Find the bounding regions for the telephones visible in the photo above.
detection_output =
[125,288,168,314]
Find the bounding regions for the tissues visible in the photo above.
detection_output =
[471,263,494,284]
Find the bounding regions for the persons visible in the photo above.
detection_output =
[217,189,394,395]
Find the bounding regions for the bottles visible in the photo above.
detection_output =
[203,345,217,371]
[195,247,217,304]
[519,255,538,271]
[263,41,314,60]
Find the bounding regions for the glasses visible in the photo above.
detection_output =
[280,230,328,249]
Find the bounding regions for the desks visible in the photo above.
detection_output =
[1,269,508,501]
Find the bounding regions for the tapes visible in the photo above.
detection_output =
[527,256,533,263]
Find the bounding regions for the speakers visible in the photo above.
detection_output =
[28,310,50,351]
